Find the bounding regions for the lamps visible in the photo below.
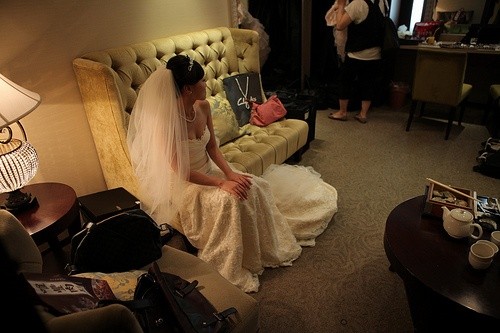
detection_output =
[0,73,42,213]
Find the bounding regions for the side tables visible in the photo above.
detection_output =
[0,183,81,266]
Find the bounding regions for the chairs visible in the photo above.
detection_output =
[406,47,472,140]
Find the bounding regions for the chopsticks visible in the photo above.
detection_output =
[425,177,483,202]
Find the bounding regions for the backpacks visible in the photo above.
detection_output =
[97,261,237,333]
[380,17,401,54]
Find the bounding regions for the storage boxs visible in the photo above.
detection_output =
[424,183,472,218]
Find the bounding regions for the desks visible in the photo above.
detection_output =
[401,45,500,54]
[384,195,500,333]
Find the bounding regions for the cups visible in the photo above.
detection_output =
[427,37,435,45]
[468,231,500,271]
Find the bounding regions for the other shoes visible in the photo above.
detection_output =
[353,113,366,122]
[328,112,347,121]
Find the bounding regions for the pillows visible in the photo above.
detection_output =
[206,91,252,146]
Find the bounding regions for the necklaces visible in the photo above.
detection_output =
[178,105,197,123]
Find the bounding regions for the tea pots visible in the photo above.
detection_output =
[441,205,483,239]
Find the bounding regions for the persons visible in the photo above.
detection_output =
[127,54,302,293]
[328,0,389,123]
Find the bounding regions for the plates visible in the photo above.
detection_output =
[476,195,500,216]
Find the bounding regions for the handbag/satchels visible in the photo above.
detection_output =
[249,94,289,126]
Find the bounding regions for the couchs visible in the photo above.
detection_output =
[72,26,308,255]
[0,209,259,333]
